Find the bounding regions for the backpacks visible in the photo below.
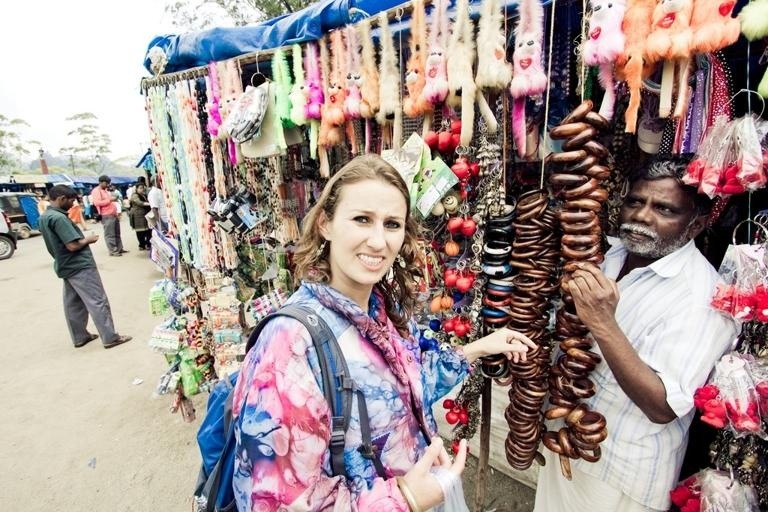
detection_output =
[194,306,352,511]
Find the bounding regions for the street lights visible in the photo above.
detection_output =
[38,147,49,175]
[64,155,74,176]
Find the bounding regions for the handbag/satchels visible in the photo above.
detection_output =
[225,86,270,143]
[93,213,102,220]
[206,185,269,234]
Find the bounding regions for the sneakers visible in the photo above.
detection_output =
[109,246,150,256]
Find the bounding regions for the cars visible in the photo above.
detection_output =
[0,192,48,261]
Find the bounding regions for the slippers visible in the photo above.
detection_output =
[75,336,97,346]
[104,336,132,347]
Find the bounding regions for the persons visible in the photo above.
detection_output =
[38,184,133,350]
[130,182,152,250]
[534,151,737,510]
[230,154,539,511]
[91,175,130,257]
[66,183,124,231]
[39,189,51,216]
[147,173,168,237]
[125,182,135,208]
